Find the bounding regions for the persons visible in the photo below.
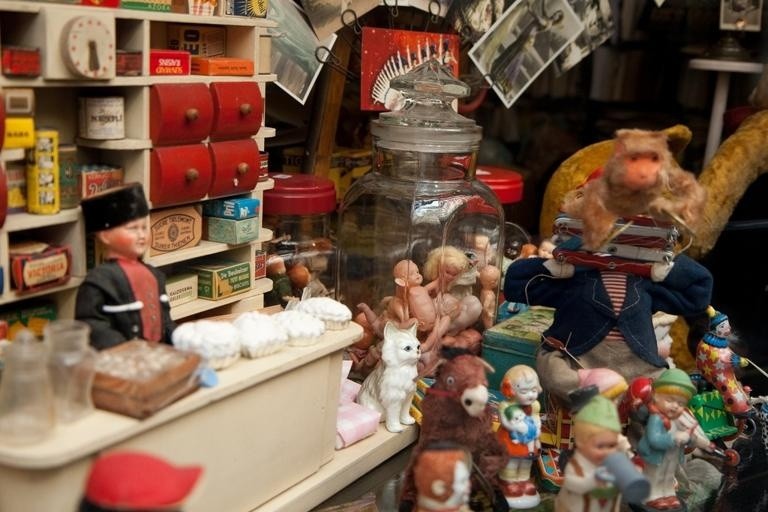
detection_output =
[354,239,754,511]
[74,180,175,350]
[580,1,598,28]
[490,1,563,85]
[354,239,755,510]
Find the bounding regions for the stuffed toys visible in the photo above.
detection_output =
[561,131,705,252]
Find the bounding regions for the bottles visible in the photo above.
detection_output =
[334,55,504,380]
[27,129,60,213]
[261,170,337,302]
[59,145,82,209]
[1,331,57,446]
[44,320,96,416]
